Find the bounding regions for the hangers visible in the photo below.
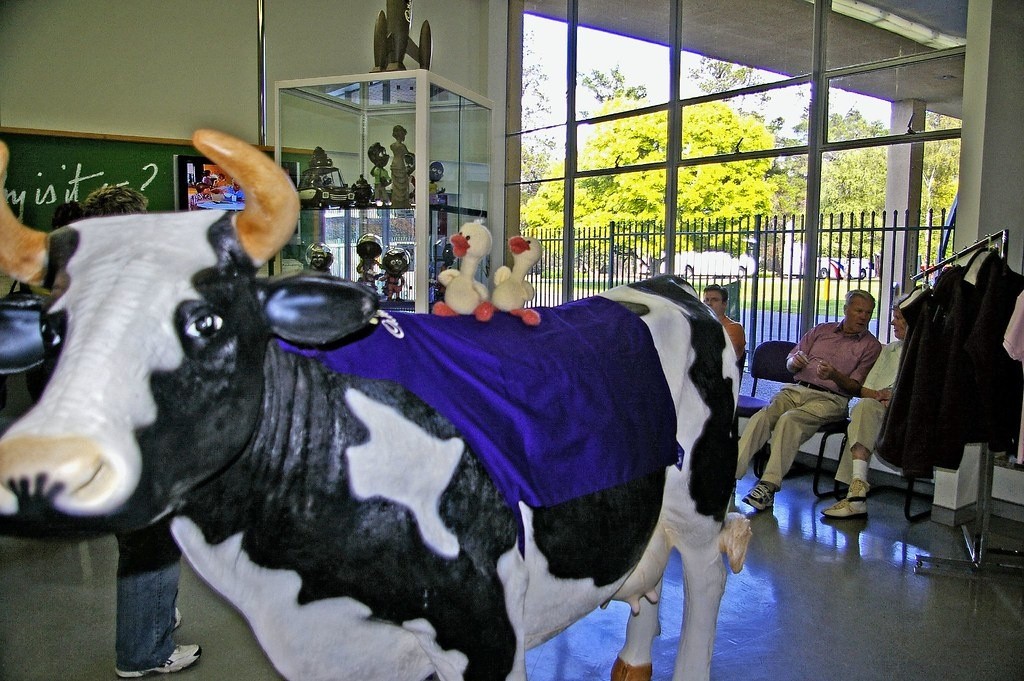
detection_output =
[919,269,925,292]
[985,235,991,254]
[951,253,958,267]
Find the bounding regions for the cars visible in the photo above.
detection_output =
[658,247,756,279]
[791,250,873,281]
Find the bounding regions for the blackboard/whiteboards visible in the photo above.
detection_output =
[0,126,326,316]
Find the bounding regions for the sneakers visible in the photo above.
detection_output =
[821,497,868,519]
[743,481,774,510]
[847,478,870,501]
[731,481,737,493]
[113,645,202,677]
[171,607,182,632]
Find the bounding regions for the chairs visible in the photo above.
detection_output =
[757,418,852,499]
[733,339,800,477]
[832,419,931,522]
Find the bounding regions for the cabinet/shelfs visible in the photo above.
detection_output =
[275,69,495,314]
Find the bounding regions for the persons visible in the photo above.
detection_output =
[822,293,914,517]
[190,169,246,204]
[39,185,203,678]
[356,233,382,290]
[726,290,882,508]
[356,173,368,185]
[378,247,409,301]
[430,161,443,203]
[391,125,412,209]
[304,242,334,276]
[368,143,392,205]
[702,284,746,366]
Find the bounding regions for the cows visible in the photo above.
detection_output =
[0,129,753,681]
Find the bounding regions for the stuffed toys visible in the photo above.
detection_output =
[490,235,542,326]
[430,223,491,321]
